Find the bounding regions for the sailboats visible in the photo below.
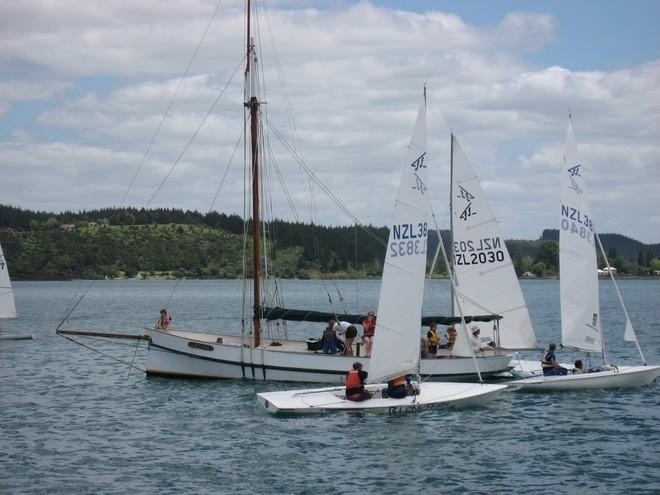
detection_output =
[508,109,659,394]
[254,83,508,417]
[0,246,33,343]
[59,0,511,381]
[440,129,576,376]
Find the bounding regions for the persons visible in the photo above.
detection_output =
[470,325,481,357]
[321,311,377,357]
[344,362,371,401]
[427,323,457,359]
[541,343,567,376]
[573,359,600,375]
[154,308,172,332]
[382,374,420,398]
[479,341,497,352]
